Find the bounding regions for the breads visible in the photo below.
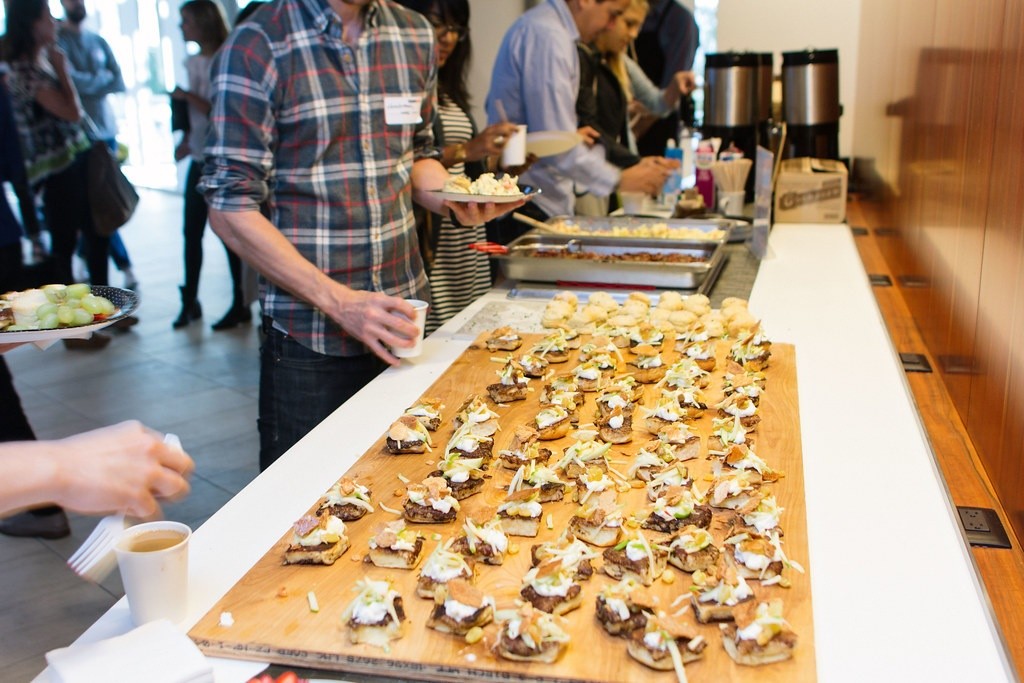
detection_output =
[543,291,758,341]
[283,327,794,670]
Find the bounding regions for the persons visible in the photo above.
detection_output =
[0,419,193,518]
[0,0,138,539]
[397,0,538,339]
[167,0,252,331]
[197,0,532,472]
[483,0,699,272]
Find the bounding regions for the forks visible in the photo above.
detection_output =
[67,433,180,577]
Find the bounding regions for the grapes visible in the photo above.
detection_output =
[36,283,116,329]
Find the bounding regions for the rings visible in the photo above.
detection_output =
[493,136,503,143]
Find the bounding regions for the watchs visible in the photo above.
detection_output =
[448,208,475,230]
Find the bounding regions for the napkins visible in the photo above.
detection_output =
[45,617,213,683]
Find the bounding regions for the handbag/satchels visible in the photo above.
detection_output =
[79,140,140,236]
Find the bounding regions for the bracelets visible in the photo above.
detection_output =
[454,143,467,160]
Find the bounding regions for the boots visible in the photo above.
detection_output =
[173,286,201,327]
[212,286,252,330]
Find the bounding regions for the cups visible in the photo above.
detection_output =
[621,190,644,215]
[499,125,528,167]
[716,189,746,216]
[113,520,192,627]
[388,299,429,358]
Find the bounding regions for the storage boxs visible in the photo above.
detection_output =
[773,156,848,223]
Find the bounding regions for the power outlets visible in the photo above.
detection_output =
[957,506,1012,549]
[899,353,933,372]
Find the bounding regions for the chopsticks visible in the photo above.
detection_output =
[708,158,753,190]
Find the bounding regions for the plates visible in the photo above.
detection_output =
[524,128,582,157]
[0,286,140,345]
[424,184,541,203]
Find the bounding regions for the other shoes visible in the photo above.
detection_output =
[0,508,70,539]
[114,316,139,328]
[63,332,111,350]
[124,272,137,290]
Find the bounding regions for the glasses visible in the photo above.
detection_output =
[429,20,470,41]
